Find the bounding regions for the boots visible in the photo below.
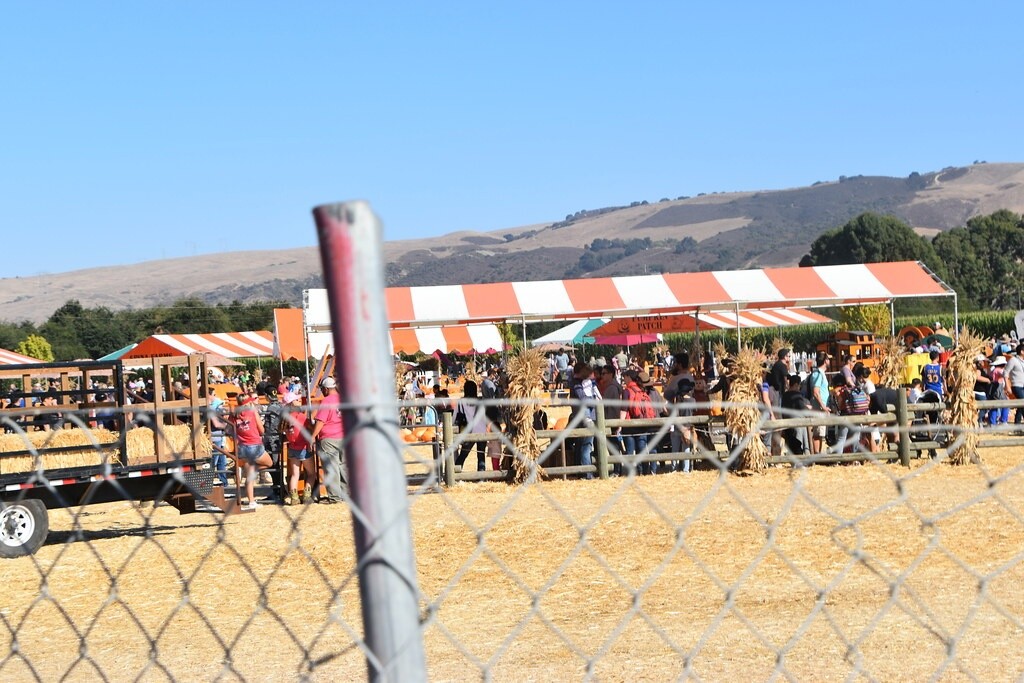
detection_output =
[302,489,314,504]
[292,492,304,505]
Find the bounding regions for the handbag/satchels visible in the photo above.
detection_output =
[454,402,467,426]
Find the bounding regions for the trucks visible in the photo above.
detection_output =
[1,360,214,559]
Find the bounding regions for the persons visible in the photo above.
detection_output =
[0,373,192,434]
[196,368,351,509]
[399,321,1024,487]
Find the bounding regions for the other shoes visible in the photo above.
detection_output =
[235,467,241,483]
[249,501,264,509]
[320,496,341,505]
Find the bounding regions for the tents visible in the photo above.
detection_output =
[96,330,274,381]
[0,348,48,364]
[272,260,959,501]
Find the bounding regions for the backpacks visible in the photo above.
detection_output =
[627,384,654,420]
[806,370,821,400]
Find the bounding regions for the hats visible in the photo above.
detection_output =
[677,379,694,395]
[211,398,225,408]
[974,355,987,360]
[239,394,258,404]
[321,378,337,388]
[639,372,654,387]
[990,356,1008,366]
[265,387,279,401]
